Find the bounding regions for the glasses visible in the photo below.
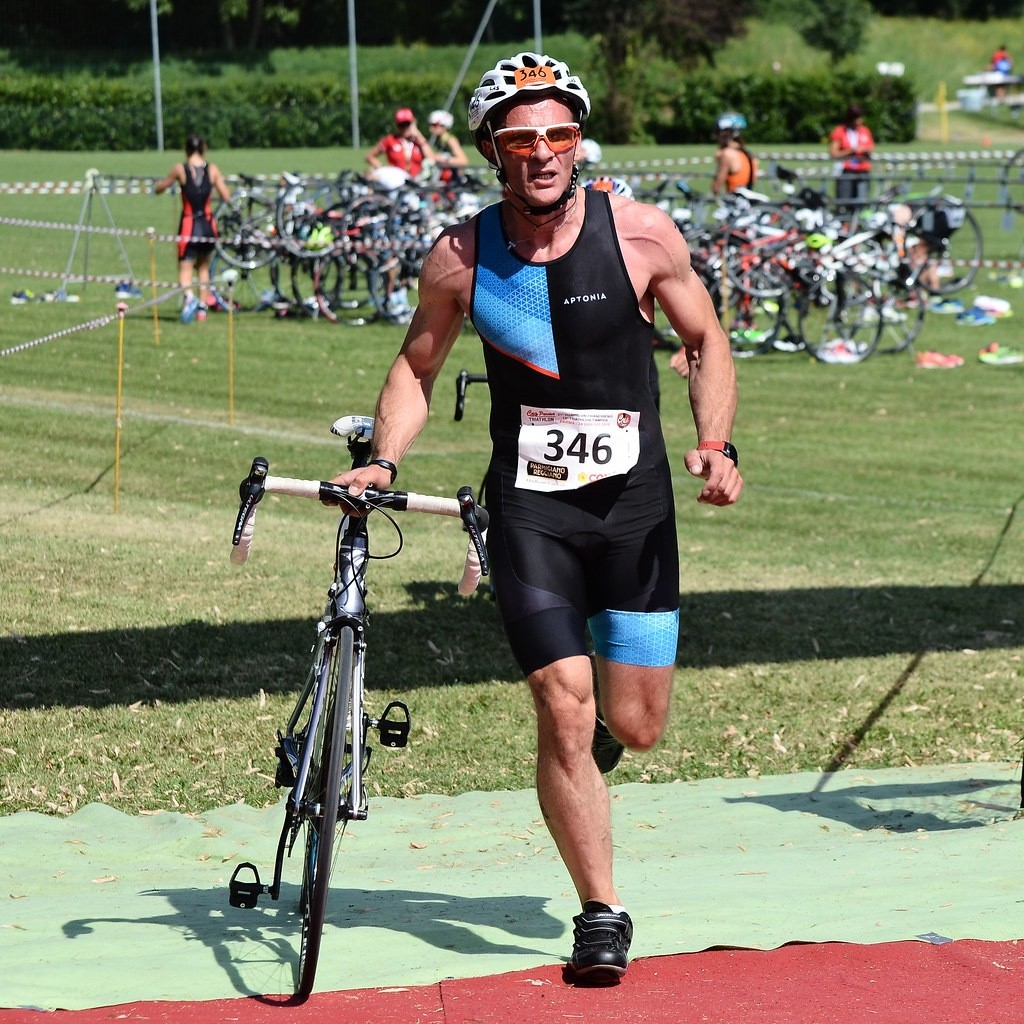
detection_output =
[488,122,580,154]
[395,121,413,126]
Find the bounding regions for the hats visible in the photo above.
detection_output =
[395,109,414,123]
[846,103,864,121]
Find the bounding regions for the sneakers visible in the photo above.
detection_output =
[729,288,1024,369]
[116,281,143,299]
[567,900,634,977]
[588,649,625,774]
[9,289,40,305]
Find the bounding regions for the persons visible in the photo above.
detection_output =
[988,46,1013,98]
[428,111,469,200]
[868,198,943,306]
[157,139,231,322]
[366,109,431,201]
[715,115,756,330]
[321,52,742,973]
[830,105,874,230]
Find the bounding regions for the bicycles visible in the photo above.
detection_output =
[208,165,987,370]
[228,413,492,1003]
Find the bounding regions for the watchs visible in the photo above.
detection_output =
[696,441,738,468]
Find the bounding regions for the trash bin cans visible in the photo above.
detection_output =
[961,90,985,113]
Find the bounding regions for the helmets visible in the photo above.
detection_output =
[428,110,455,129]
[717,113,747,130]
[580,139,603,162]
[467,52,591,163]
[586,175,635,200]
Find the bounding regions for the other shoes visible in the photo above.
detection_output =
[182,294,198,324]
[196,301,206,319]
[42,288,81,304]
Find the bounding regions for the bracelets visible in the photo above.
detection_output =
[365,460,398,483]
